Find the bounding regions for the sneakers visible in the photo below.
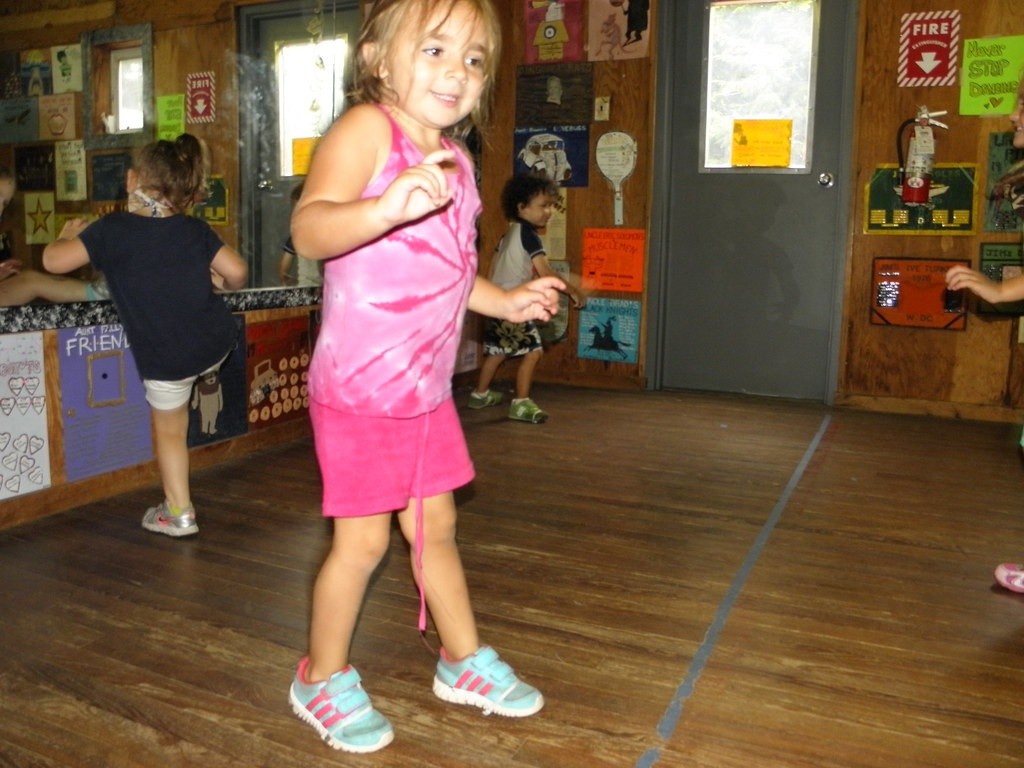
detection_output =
[289,657,395,754]
[432,645,544,718]
[142,499,199,536]
[468,388,504,409]
[508,398,548,424]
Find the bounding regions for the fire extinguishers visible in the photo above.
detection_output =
[896,102,950,207]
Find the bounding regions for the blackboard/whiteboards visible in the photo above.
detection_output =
[79,147,136,208]
[10,141,58,193]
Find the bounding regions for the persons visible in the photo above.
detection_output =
[0,166,111,307]
[42,133,248,537]
[942,75,1024,596]
[289,0,569,752]
[466,165,585,425]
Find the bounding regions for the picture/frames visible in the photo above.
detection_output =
[862,161,979,236]
[185,176,229,227]
[896,8,962,89]
[869,256,972,332]
[977,243,1024,318]
[186,71,217,125]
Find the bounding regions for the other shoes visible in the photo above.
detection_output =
[994,563,1024,593]
[92,273,111,301]
